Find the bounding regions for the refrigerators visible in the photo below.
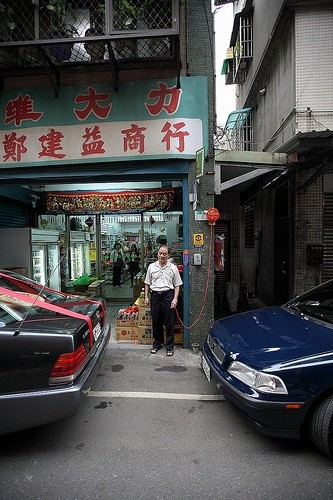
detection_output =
[0,227,61,292]
[69,231,91,280]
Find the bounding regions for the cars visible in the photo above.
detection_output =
[200,278,333,460]
[0,267,111,437]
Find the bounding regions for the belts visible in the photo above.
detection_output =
[153,290,167,294]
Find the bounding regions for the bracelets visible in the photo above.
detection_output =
[173,299,178,303]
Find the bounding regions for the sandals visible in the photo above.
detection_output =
[150,346,159,354]
[166,350,174,356]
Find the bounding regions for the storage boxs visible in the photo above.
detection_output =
[88,280,106,298]
[114,283,184,344]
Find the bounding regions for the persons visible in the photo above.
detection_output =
[108,235,167,289]
[144,244,183,356]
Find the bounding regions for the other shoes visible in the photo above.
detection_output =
[119,285,122,288]
[113,286,118,288]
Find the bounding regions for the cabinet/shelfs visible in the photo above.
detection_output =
[89,233,139,284]
